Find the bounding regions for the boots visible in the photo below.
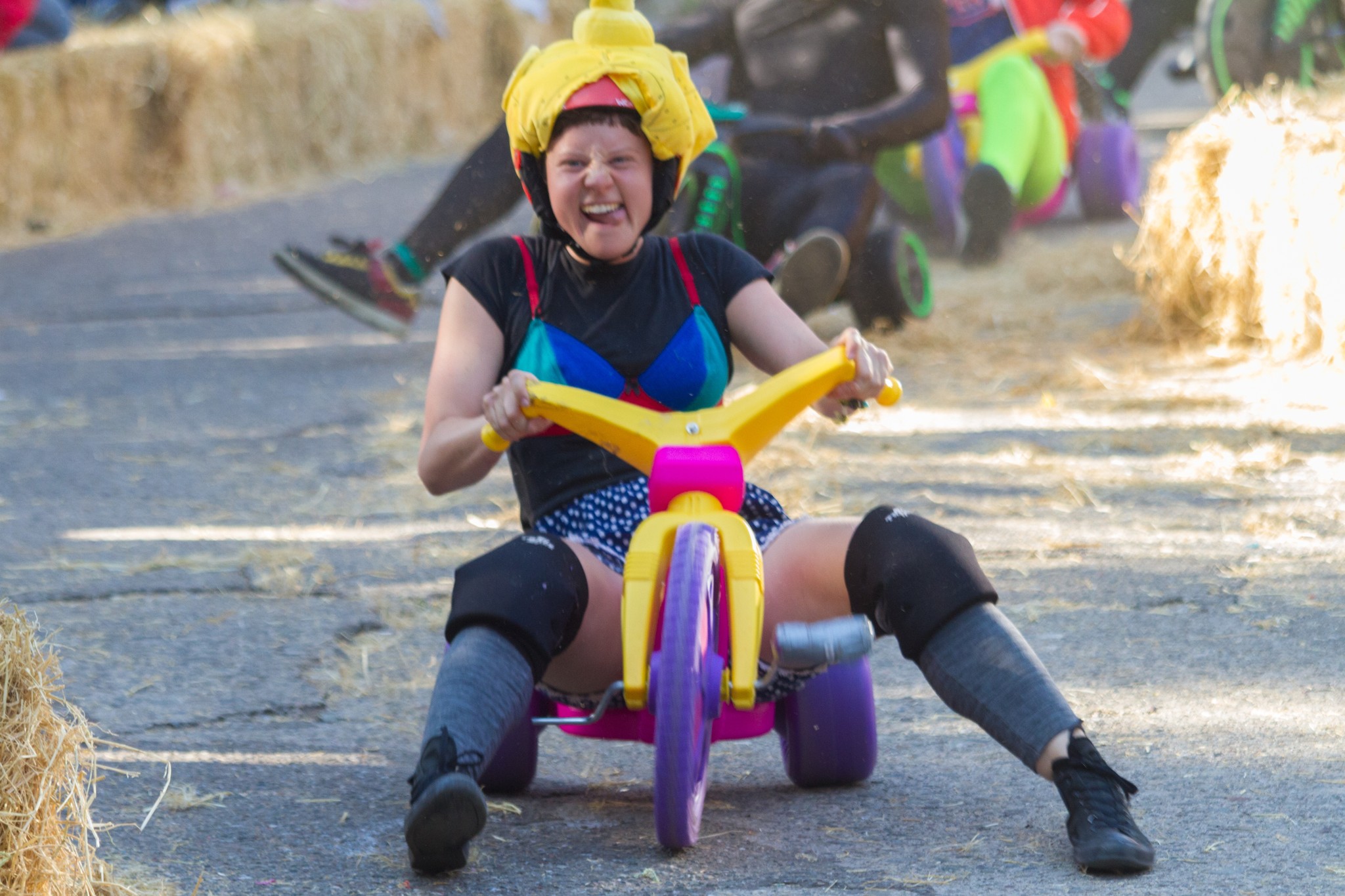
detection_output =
[1053,737,1155,869]
[406,727,487,874]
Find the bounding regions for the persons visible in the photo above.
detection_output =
[393,26,1164,877]
[242,0,1345,337]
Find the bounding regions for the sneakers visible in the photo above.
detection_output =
[274,246,420,335]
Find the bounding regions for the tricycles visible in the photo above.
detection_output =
[592,89,939,336]
[448,334,910,844]
[1167,1,1345,118]
[824,20,1148,264]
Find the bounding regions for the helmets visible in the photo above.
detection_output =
[513,42,684,260]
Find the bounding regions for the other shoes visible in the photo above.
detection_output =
[771,231,847,317]
[1093,76,1129,126]
[964,163,1013,265]
[329,233,374,257]
